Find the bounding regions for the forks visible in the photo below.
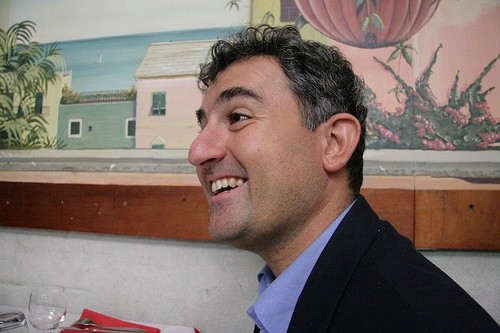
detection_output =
[71,318,147,332]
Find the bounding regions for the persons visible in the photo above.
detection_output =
[188,25,500,333]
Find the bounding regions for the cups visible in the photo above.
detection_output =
[0,311,28,333]
[29,286,67,333]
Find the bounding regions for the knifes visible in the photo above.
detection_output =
[59,327,140,333]
